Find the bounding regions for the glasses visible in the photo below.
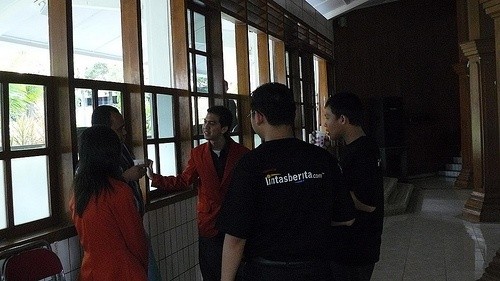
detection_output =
[247,109,263,118]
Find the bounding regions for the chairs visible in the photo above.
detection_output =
[0,240,67,281]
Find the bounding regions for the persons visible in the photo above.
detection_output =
[67,127,150,281]
[89,106,161,280]
[218,82,358,280]
[321,91,384,280]
[146,106,266,281]
